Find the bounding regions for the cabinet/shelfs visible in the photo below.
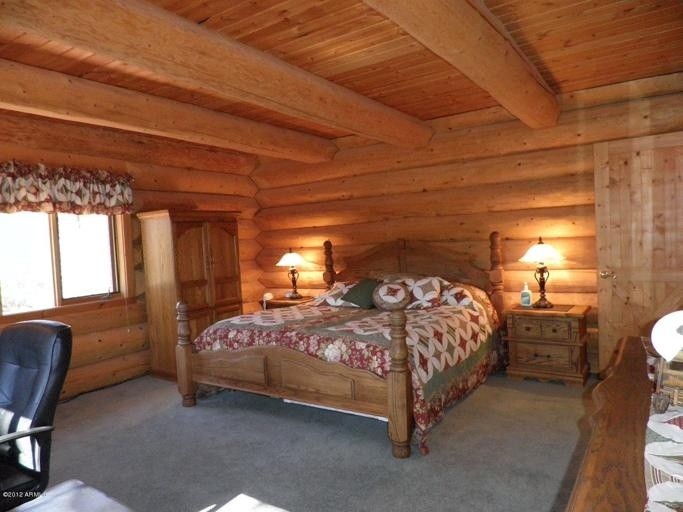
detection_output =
[136,205,244,383]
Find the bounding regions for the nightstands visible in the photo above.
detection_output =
[501,302,593,389]
[259,294,316,311]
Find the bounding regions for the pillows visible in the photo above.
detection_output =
[311,273,473,314]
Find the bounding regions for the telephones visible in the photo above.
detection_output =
[264,292,273,301]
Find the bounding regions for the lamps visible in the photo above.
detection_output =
[517,234,567,309]
[275,247,311,301]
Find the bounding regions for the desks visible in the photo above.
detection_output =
[545,332,683,512]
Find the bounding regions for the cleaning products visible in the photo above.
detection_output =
[520,283,531,307]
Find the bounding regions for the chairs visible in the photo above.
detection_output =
[0,319,74,511]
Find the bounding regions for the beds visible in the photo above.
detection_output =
[172,228,504,460]
